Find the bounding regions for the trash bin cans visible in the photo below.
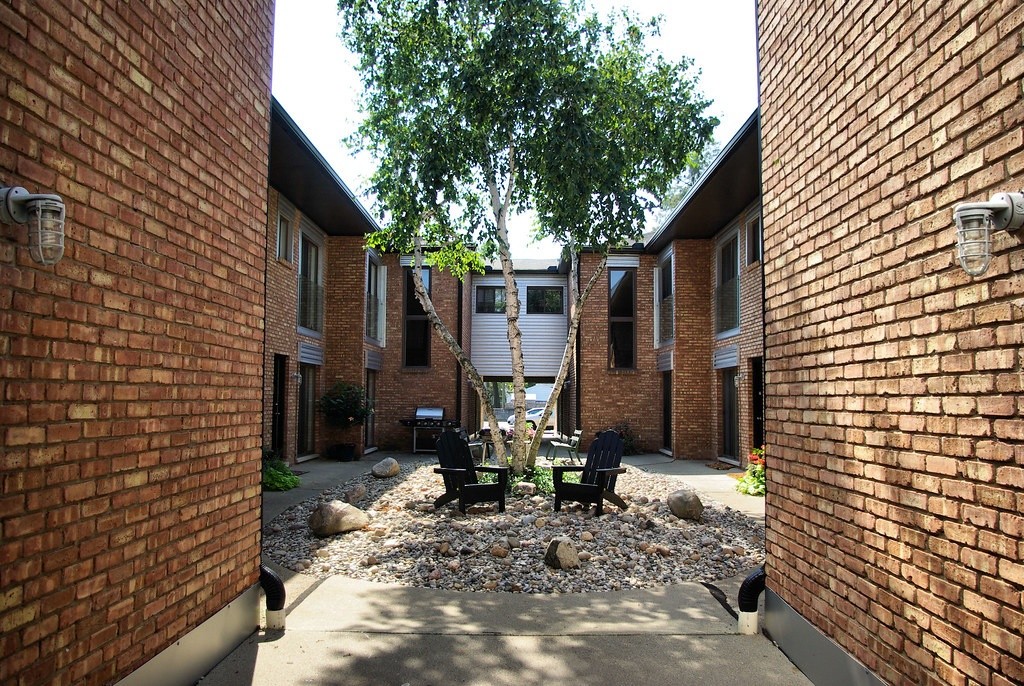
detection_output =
[413,406,446,453]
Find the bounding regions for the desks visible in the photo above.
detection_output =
[484,440,531,461]
[413,426,448,453]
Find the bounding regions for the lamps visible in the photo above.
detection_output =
[564,381,570,389]
[733,374,744,387]
[291,373,302,385]
[0,186,65,267]
[952,191,1024,277]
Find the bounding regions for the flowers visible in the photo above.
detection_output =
[311,381,375,445]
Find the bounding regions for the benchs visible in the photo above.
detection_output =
[453,426,485,459]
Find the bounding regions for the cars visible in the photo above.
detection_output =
[506,407,544,425]
[525,408,555,431]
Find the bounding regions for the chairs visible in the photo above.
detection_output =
[546,428,583,465]
[551,429,629,517]
[433,431,510,515]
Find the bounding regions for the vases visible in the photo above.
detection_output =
[332,444,356,462]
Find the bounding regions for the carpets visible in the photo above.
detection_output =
[705,461,735,470]
